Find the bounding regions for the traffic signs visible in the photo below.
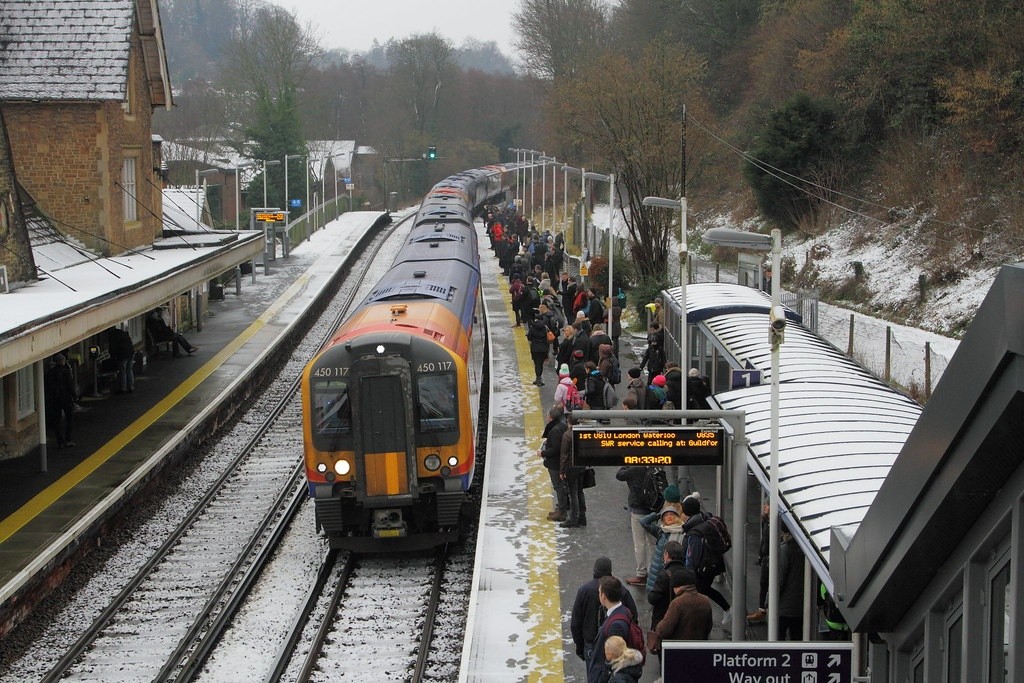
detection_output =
[661,639,855,683]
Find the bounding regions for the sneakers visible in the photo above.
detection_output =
[722,606,733,625]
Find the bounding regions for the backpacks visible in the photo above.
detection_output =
[559,382,582,412]
[603,606,646,666]
[595,375,618,408]
[630,465,668,512]
[689,518,733,556]
[514,285,525,301]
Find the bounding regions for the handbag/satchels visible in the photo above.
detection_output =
[582,468,596,489]
[617,288,626,308]
[544,325,556,343]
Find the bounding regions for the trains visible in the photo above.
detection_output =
[301,161,550,553]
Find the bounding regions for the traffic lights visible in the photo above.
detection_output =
[428,147,436,159]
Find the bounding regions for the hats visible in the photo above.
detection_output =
[663,483,680,503]
[653,375,666,386]
[682,497,700,517]
[628,367,641,378]
[559,363,570,378]
[577,311,585,319]
[671,570,695,588]
[593,557,612,575]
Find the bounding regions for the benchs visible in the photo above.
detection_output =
[147,325,185,353]
[96,346,138,390]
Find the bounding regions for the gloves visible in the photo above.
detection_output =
[575,641,585,661]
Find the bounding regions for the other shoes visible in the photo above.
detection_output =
[622,573,646,586]
[555,502,570,511]
[567,516,586,526]
[559,519,578,527]
[533,379,545,386]
[547,510,567,521]
[747,610,766,622]
[602,419,610,423]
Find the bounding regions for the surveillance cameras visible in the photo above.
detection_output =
[679,243,688,259]
[770,305,786,331]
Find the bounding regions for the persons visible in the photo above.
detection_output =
[48,356,77,448]
[571,556,647,683]
[763,268,771,295]
[746,498,852,643]
[622,320,711,425]
[605,635,644,683]
[149,307,199,358]
[653,298,664,323]
[109,329,136,392]
[616,465,734,683]
[482,202,626,424]
[588,576,632,683]
[536,403,587,528]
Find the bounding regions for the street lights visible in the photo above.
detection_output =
[323,151,360,228]
[306,157,319,241]
[539,156,556,244]
[263,160,281,252]
[548,162,567,253]
[236,162,255,231]
[285,154,301,258]
[700,228,782,641]
[508,148,545,235]
[584,172,615,344]
[642,195,688,499]
[196,169,219,230]
[561,167,585,284]
